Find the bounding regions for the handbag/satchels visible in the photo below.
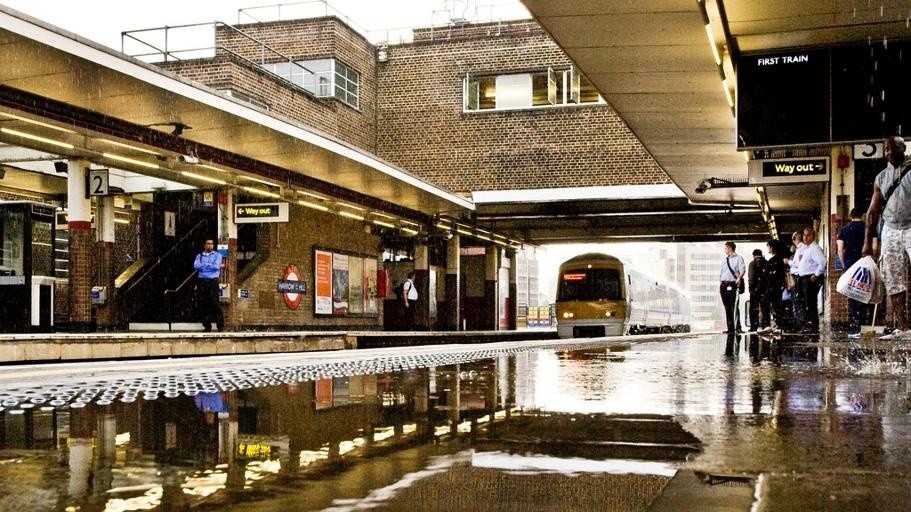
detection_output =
[739,278,745,294]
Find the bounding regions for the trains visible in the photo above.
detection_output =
[553,250,691,339]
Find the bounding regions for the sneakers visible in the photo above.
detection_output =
[722,327,784,336]
[847,326,862,338]
[878,329,911,342]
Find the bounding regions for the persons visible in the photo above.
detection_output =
[195,391,224,424]
[403,272,418,331]
[836,207,878,334]
[194,239,225,332]
[747,226,827,333]
[862,136,911,340]
[720,242,745,333]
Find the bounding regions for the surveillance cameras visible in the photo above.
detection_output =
[177,153,200,164]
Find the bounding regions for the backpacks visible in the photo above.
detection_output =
[399,280,412,294]
